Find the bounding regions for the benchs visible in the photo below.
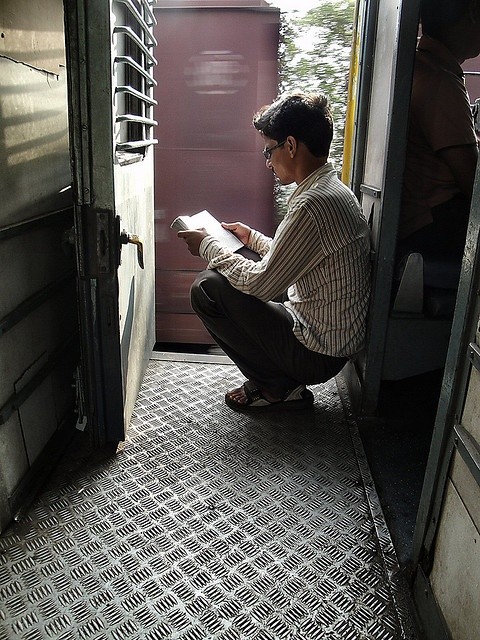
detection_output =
[379,253,461,381]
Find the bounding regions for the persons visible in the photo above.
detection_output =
[177,92,375,414]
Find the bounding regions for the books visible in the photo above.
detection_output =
[170,210,245,254]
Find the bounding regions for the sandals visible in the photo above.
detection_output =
[225,377,314,412]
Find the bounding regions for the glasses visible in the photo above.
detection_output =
[262,139,286,159]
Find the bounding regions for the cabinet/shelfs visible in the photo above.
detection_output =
[407,153,479,639]
